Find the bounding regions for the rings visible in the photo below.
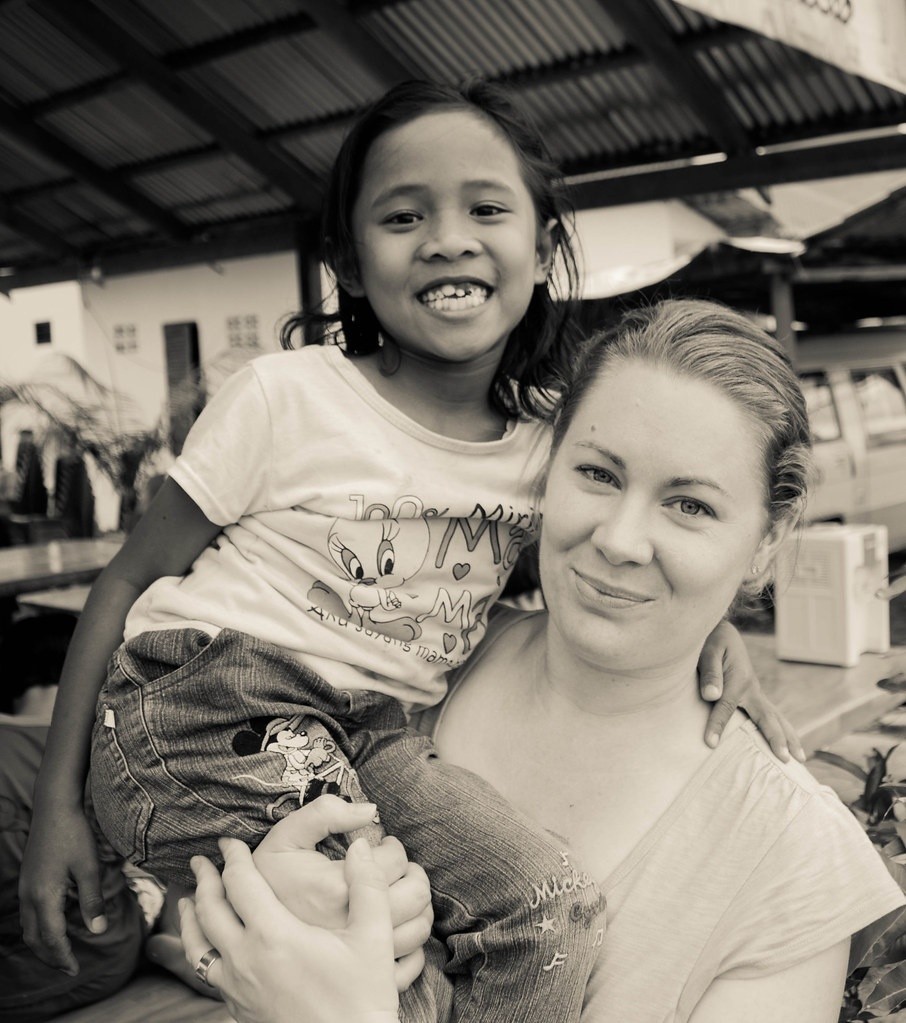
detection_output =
[193,948,221,988]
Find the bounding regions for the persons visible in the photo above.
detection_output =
[14,78,806,1023]
[151,296,906,1023]
